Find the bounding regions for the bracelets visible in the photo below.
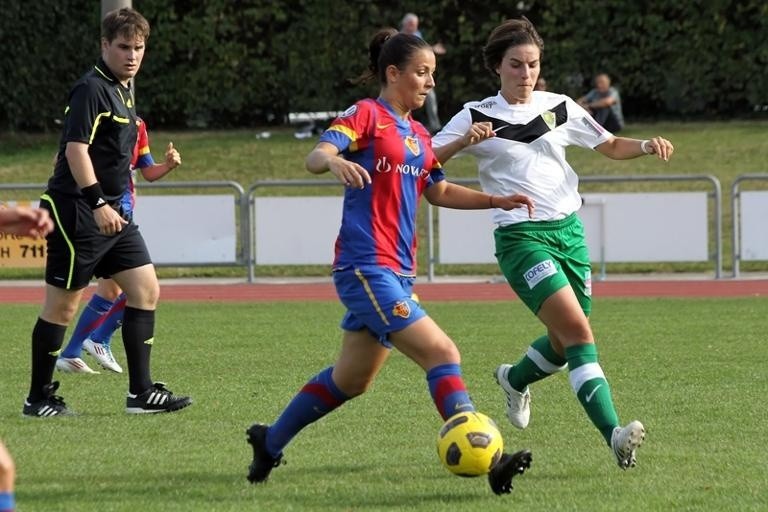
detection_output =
[641,138,652,154]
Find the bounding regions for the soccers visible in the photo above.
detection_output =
[436,410,505,478]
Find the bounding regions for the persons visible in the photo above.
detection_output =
[55,114,182,372]
[245,29,532,495]
[428,17,674,470]
[0,196,57,511]
[531,71,549,93]
[21,7,193,415]
[575,73,626,133]
[394,12,447,136]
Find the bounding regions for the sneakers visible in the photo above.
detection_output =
[245,423,284,483]
[83,338,123,373]
[22,396,75,418]
[611,420,646,471]
[56,353,100,379]
[493,363,531,429]
[126,383,193,415]
[488,449,533,495]
